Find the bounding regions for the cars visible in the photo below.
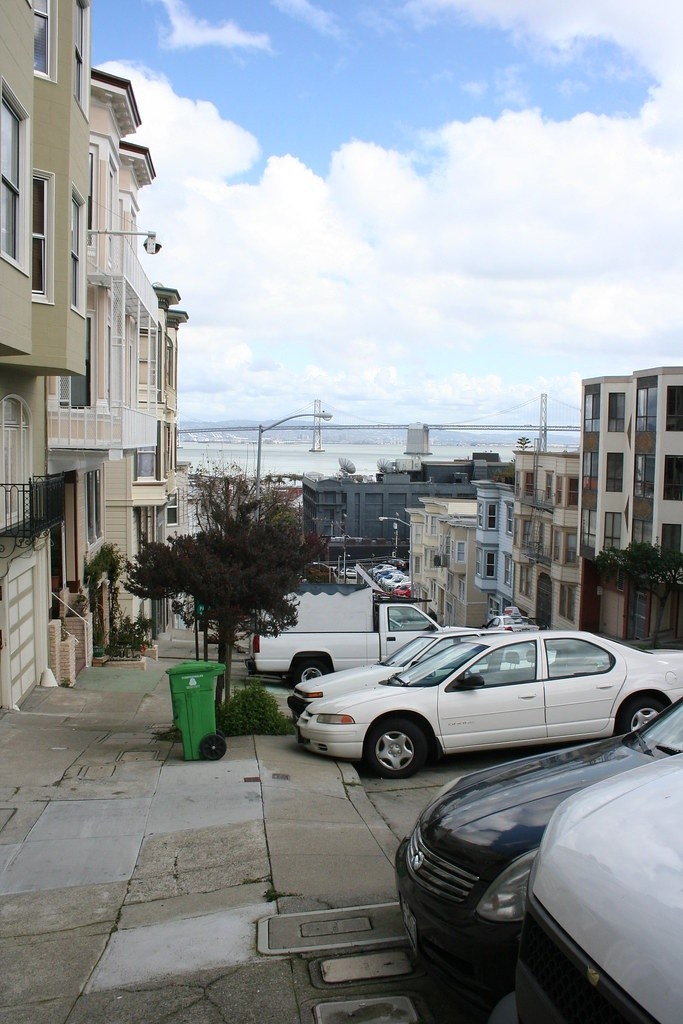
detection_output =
[293,628,682,779]
[481,607,540,632]
[368,564,412,597]
[311,562,338,575]
[335,566,356,579]
[394,696,683,1024]
[287,629,559,721]
[382,558,406,570]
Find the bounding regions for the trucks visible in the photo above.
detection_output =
[244,583,486,687]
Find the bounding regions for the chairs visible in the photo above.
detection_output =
[498,650,536,683]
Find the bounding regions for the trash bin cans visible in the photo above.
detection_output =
[163,660,229,763]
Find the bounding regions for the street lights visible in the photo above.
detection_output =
[344,534,352,582]
[255,411,333,521]
[379,517,412,579]
[337,555,341,580]
